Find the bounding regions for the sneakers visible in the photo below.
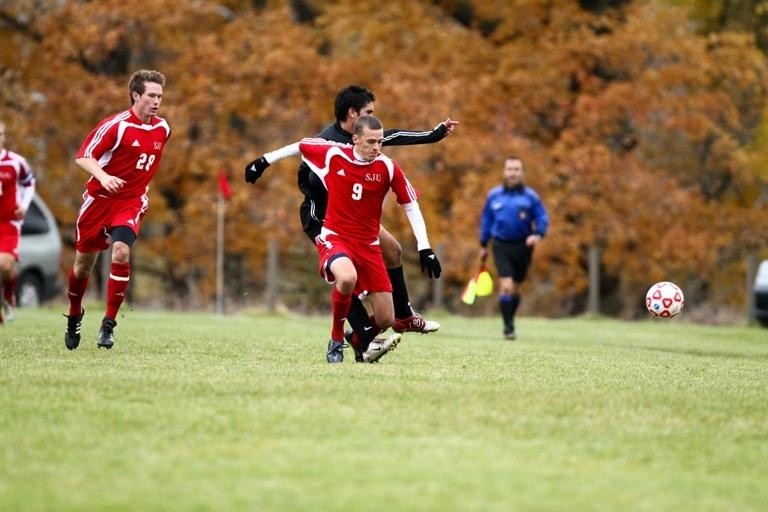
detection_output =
[97,318,117,350]
[394,311,439,333]
[326,325,402,363]
[504,323,515,338]
[2,299,15,322]
[62,307,84,350]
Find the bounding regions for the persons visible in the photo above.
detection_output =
[475,157,551,343]
[299,84,460,363]
[243,116,442,364]
[63,68,173,353]
[0,124,36,329]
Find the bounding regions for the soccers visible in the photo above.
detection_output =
[645,281,684,318]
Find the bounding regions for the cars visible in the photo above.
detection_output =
[751,258,768,328]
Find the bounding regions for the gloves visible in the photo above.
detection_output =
[245,157,270,184]
[419,249,441,278]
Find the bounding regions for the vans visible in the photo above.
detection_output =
[12,188,62,311]
